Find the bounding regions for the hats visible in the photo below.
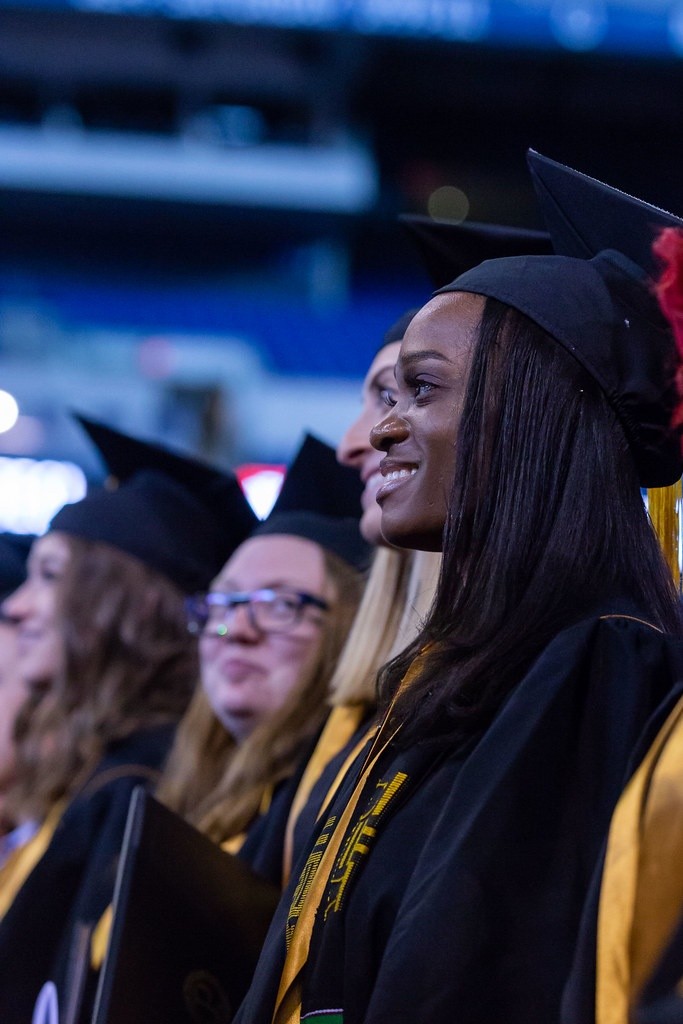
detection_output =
[435,148,683,487]
[255,429,375,574]
[48,405,261,594]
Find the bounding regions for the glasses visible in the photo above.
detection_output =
[185,584,331,634]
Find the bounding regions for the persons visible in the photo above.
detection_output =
[0,327,448,1024]
[229,148,683,1024]
